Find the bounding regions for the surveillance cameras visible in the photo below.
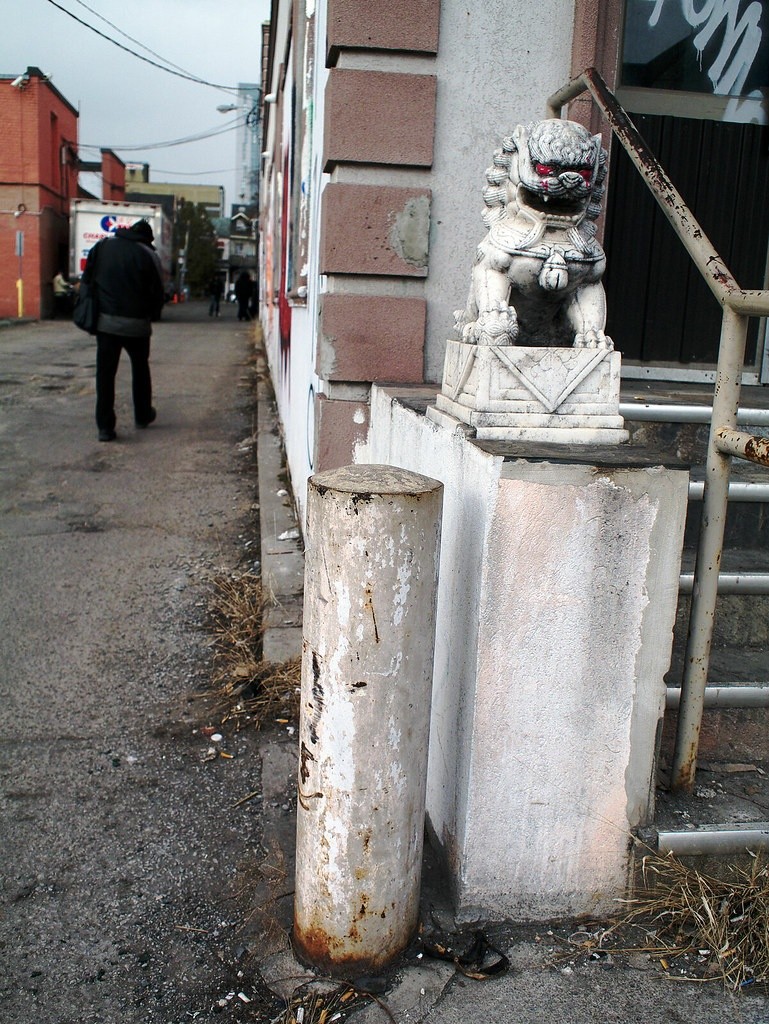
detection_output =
[11,76,23,87]
[43,73,53,79]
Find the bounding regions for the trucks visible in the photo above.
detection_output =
[64,195,186,306]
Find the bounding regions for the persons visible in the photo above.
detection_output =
[51,219,166,442]
[207,271,259,323]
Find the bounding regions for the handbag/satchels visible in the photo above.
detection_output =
[73,237,108,335]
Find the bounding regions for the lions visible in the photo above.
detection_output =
[444,118,614,351]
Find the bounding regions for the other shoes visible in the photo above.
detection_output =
[136,407,156,429]
[99,428,117,441]
